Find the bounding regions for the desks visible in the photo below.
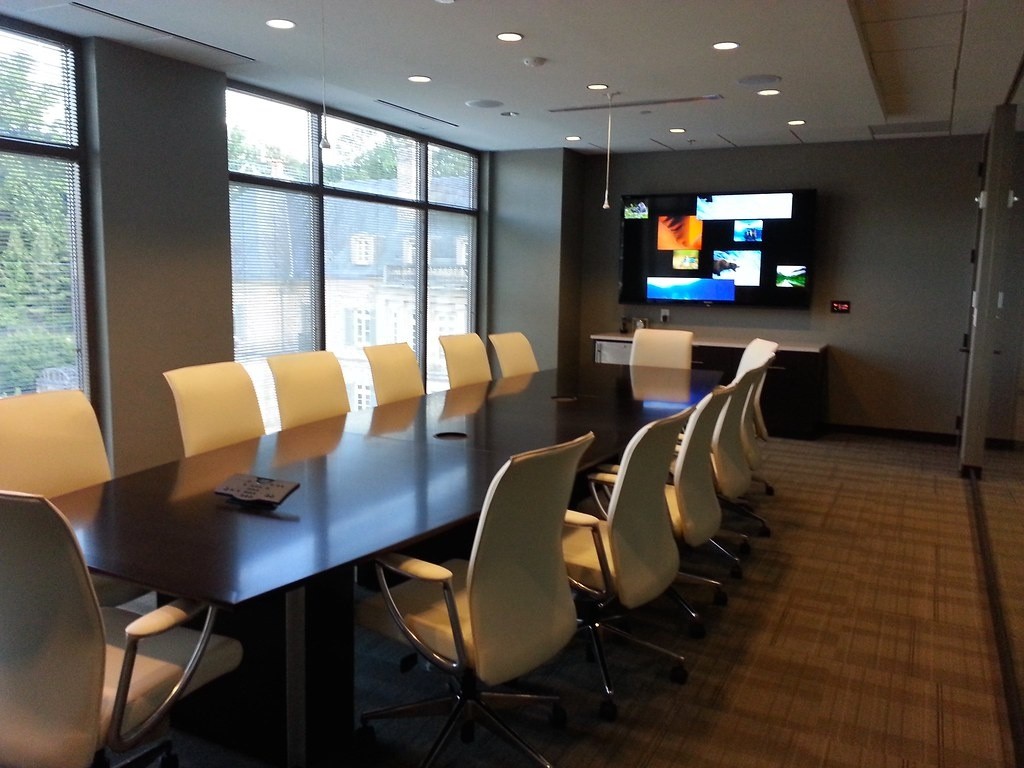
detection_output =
[45,362,724,768]
[590,329,831,437]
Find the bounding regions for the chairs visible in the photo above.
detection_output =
[0,327,779,768]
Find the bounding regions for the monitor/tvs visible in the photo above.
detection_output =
[619,188,821,309]
